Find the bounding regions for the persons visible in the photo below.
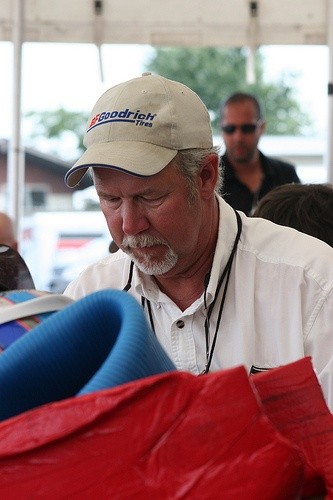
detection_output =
[213,93,301,216]
[250,184,333,249]
[63,71,333,420]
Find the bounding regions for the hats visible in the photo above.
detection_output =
[64,72,213,188]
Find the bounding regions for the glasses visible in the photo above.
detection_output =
[220,123,257,134]
